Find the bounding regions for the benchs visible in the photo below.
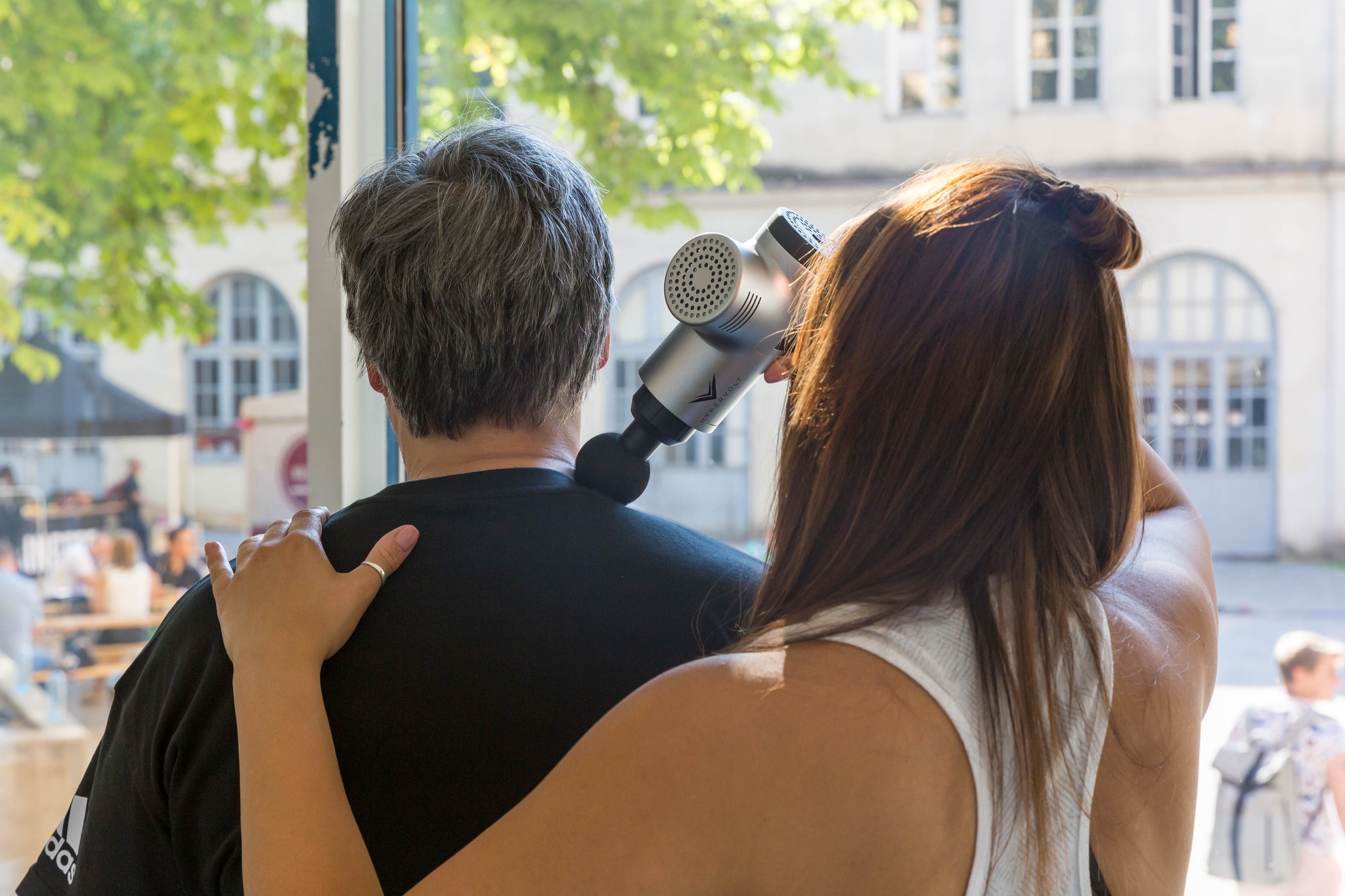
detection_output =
[34,640,153,683]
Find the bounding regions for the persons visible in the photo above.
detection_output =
[117,458,154,565]
[203,166,1216,896]
[60,531,114,669]
[1,536,72,726]
[16,119,774,896]
[150,524,200,590]
[1235,630,1344,896]
[67,530,161,707]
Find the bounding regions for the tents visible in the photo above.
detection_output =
[0,333,187,528]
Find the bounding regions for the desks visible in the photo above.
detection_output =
[35,613,168,701]
[92,587,188,610]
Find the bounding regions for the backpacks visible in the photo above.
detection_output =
[1207,708,1339,884]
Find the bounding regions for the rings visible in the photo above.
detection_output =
[360,561,387,583]
